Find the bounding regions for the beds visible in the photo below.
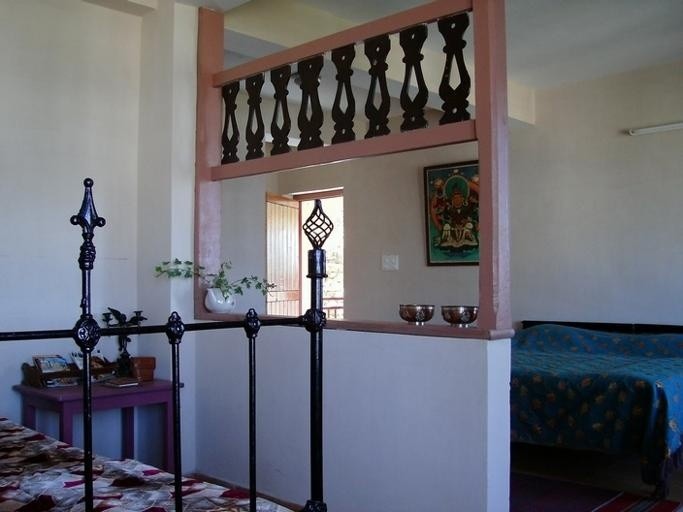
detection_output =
[510,320,683,499]
[0,177,334,511]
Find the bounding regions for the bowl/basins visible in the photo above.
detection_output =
[398,304,437,326]
[440,306,479,327]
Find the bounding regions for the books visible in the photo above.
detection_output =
[31,349,139,389]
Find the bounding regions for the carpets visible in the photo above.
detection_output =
[510,472,681,512]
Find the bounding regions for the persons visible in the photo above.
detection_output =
[429,174,480,254]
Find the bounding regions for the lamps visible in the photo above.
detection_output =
[628,122,683,136]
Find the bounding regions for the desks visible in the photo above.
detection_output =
[12,379,184,474]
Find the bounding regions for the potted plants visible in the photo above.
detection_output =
[154,255,277,315]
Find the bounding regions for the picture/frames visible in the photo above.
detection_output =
[423,160,480,266]
